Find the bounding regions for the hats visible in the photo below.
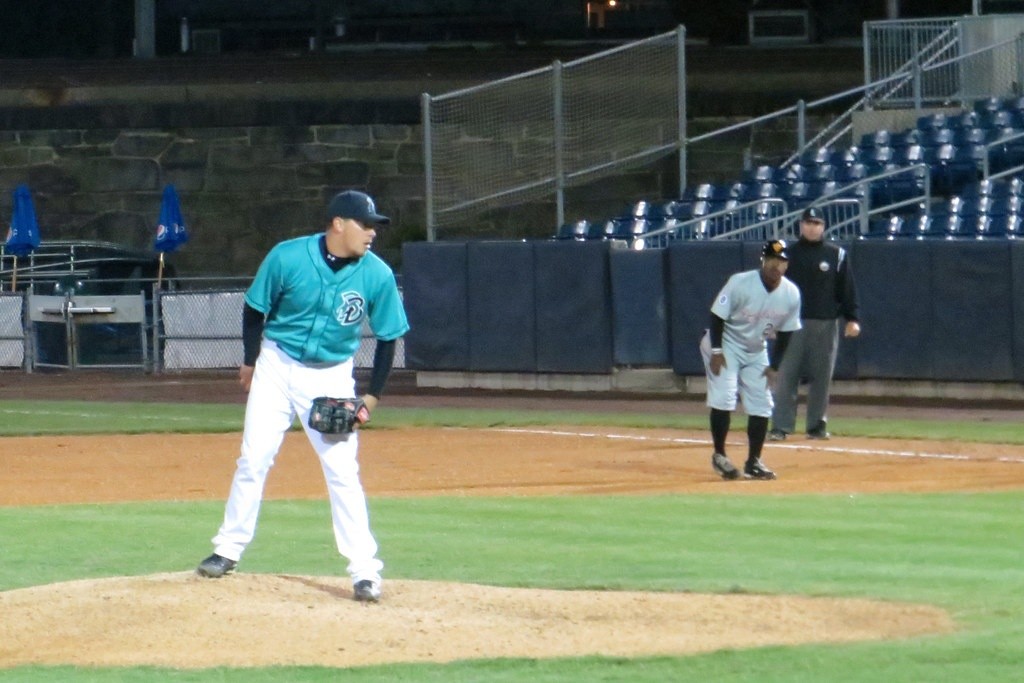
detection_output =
[327,191,391,227]
[802,207,825,224]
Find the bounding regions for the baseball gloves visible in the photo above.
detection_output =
[308,396,372,434]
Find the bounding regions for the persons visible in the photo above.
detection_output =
[767,208,862,440]
[197,190,410,601]
[700,240,803,480]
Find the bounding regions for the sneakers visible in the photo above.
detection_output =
[711,453,739,480]
[198,552,237,578]
[767,428,785,440]
[743,461,776,481]
[806,428,830,439]
[353,579,380,603]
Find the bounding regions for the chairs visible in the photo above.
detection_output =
[553,77,1024,247]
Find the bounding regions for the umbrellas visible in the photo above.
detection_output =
[6,184,40,292]
[155,183,190,292]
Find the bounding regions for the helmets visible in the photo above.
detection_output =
[761,239,789,260]
